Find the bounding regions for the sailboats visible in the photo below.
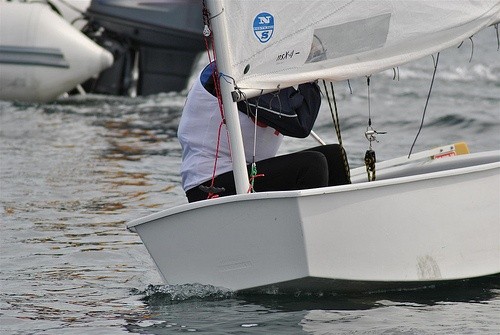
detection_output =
[126,0,500,299]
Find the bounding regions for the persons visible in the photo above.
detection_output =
[177,59,353,203]
